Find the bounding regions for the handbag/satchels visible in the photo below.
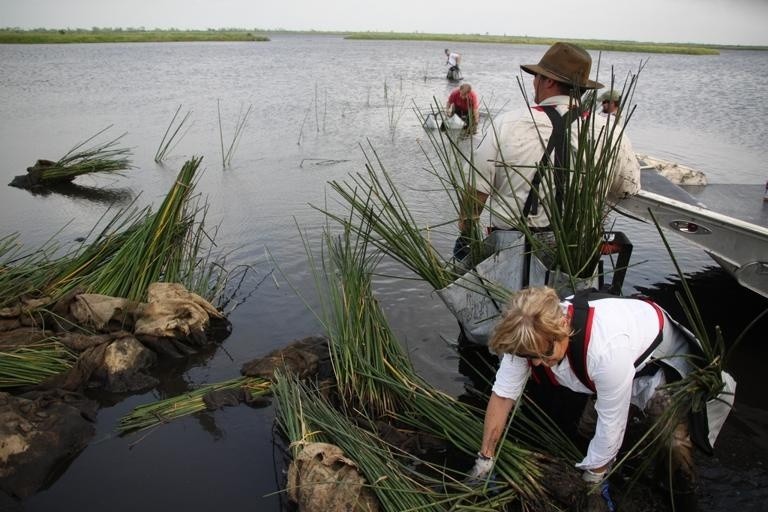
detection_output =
[653,299,737,455]
[433,230,526,345]
[529,231,600,300]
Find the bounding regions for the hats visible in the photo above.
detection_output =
[596,91,622,101]
[520,42,605,88]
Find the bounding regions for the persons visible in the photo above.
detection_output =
[445,49,461,81]
[446,84,479,133]
[464,286,723,512]
[595,90,623,125]
[454,43,641,408]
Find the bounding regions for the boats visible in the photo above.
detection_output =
[601,167,766,304]
